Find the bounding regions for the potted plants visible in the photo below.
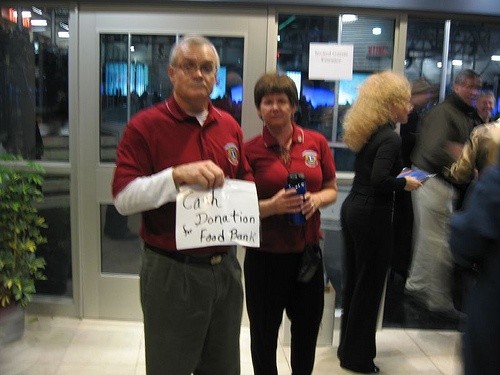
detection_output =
[0,142,50,345]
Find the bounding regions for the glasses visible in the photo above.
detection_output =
[171,60,216,77]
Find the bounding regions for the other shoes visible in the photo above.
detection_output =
[341,358,379,374]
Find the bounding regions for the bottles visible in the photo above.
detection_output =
[285,173,308,224]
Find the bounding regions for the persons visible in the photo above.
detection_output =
[104,91,162,241]
[243,74,337,375]
[210,95,242,128]
[296,96,350,130]
[113,37,255,375]
[37,90,67,192]
[449,162,500,375]
[391,71,496,323]
[337,70,423,374]
[446,116,500,212]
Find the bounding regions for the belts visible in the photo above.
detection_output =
[145,242,230,266]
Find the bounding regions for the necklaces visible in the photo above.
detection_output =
[279,130,293,159]
